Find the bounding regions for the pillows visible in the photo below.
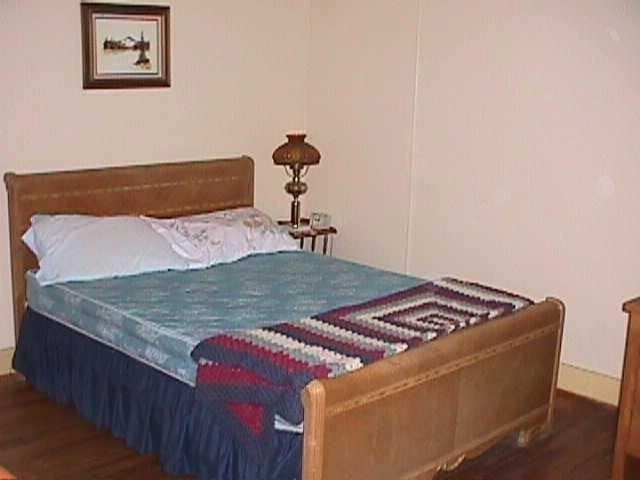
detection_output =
[20,205,304,288]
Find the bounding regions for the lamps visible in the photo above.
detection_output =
[272,134,322,228]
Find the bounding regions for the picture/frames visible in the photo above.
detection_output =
[79,1,172,91]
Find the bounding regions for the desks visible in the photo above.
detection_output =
[277,218,337,255]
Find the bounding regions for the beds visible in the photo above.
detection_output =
[4,154,565,480]
